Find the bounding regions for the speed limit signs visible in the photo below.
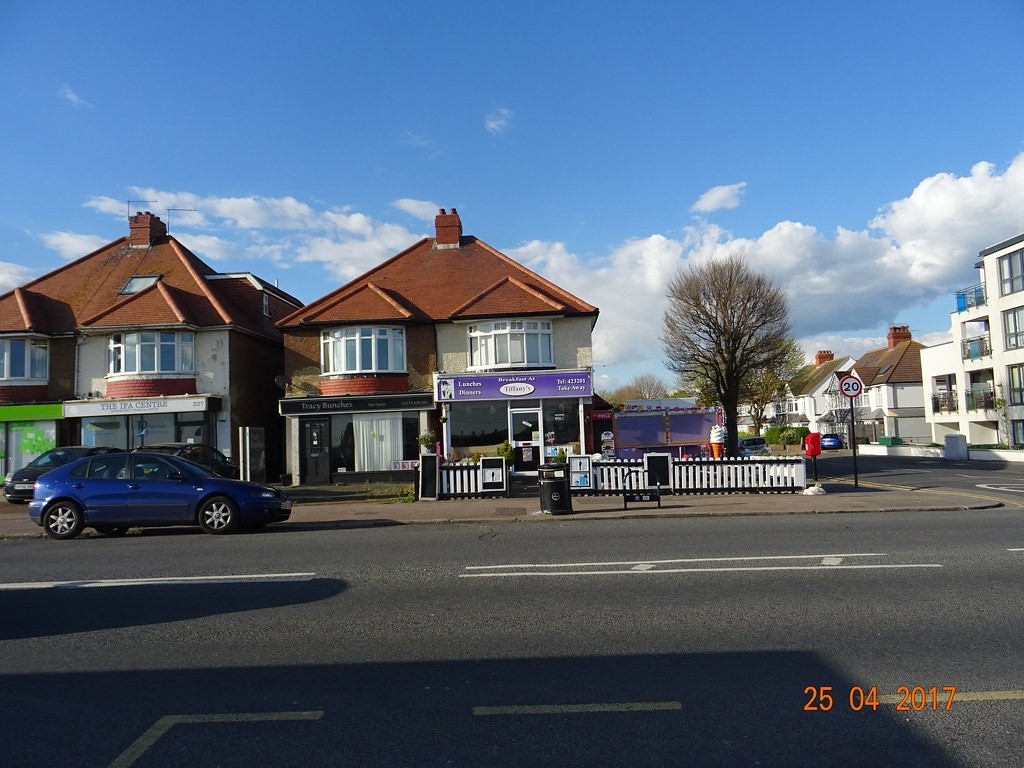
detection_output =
[839,375,862,398]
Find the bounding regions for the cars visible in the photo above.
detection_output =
[821,432,848,450]
[28,449,294,538]
[740,437,771,455]
[2,445,127,503]
[118,442,240,488]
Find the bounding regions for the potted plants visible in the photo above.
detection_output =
[416,431,436,454]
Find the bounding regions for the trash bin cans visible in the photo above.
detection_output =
[878,436,902,445]
[944,434,968,461]
[538,462,573,516]
